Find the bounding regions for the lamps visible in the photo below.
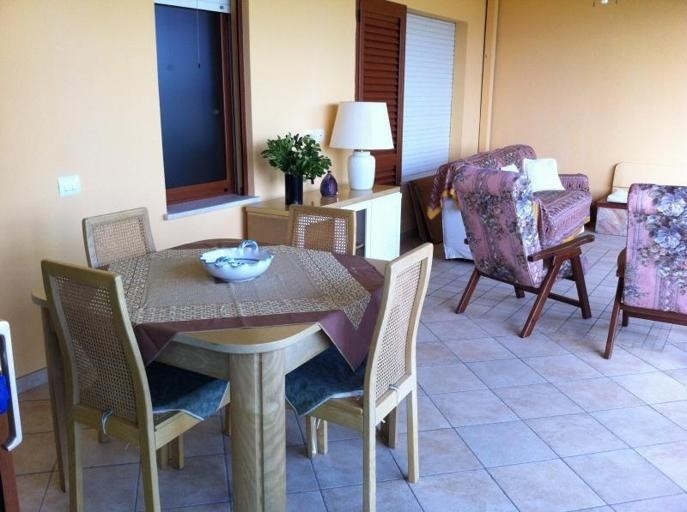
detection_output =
[328,100,396,192]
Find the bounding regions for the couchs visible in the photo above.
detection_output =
[593,162,687,237]
[426,144,593,262]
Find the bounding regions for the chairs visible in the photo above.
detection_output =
[452,164,596,340]
[40,259,230,512]
[604,183,687,360]
[285,242,434,512]
[82,206,157,268]
[286,204,357,256]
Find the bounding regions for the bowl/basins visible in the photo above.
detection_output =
[199,239,274,283]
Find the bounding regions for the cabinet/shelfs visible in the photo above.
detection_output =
[241,183,402,262]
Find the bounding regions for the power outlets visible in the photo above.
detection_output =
[315,128,324,142]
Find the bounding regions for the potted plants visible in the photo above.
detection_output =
[260,132,332,205]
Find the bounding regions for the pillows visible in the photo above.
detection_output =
[502,164,520,173]
[521,158,565,193]
